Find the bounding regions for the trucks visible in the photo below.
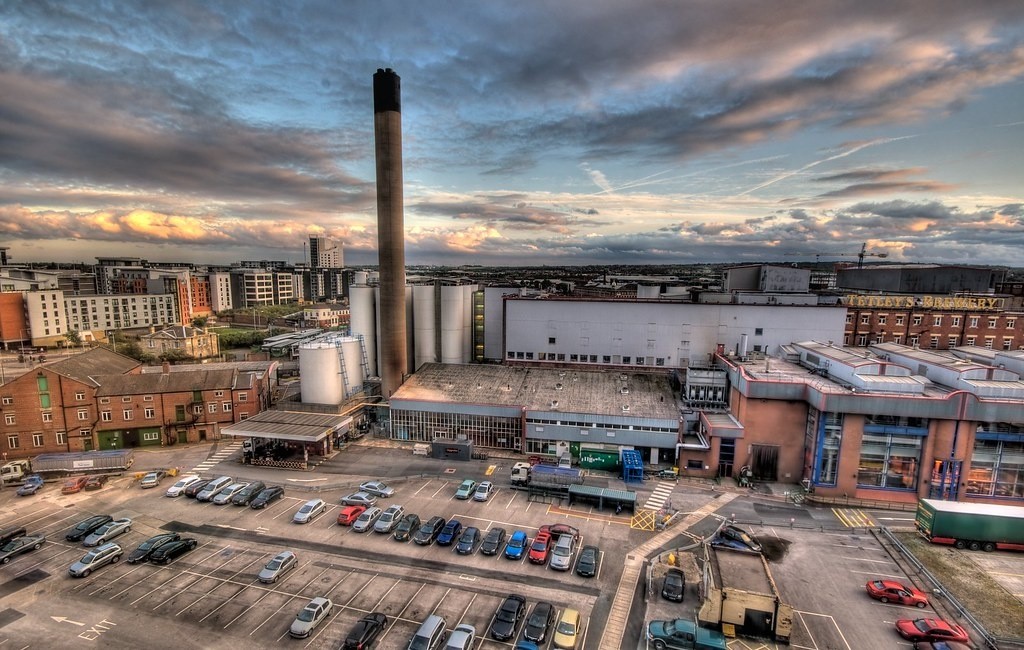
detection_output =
[645,618,726,650]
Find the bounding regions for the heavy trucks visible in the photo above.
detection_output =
[511,461,585,495]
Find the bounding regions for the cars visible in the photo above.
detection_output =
[344,612,388,650]
[866,579,929,609]
[293,479,580,572]
[258,550,298,584]
[575,545,599,578]
[441,624,477,650]
[913,640,972,650]
[524,601,557,644]
[895,617,970,643]
[720,525,762,555]
[490,594,526,642]
[554,608,581,650]
[0,469,285,579]
[661,568,686,603]
[289,596,333,638]
[406,614,447,650]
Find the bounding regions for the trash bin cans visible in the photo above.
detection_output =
[673,468,679,475]
